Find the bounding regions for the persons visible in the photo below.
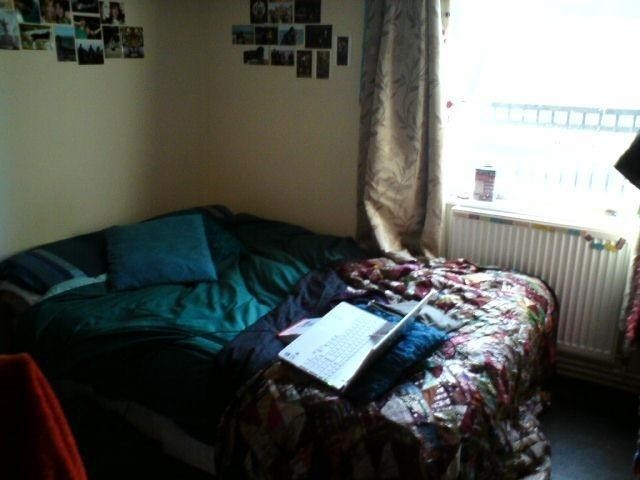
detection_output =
[255,6,310,73]
[41,0,122,63]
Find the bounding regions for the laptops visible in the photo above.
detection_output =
[277,288,435,393]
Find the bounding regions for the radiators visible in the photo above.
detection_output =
[448,205,630,363]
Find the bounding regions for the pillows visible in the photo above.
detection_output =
[0,205,243,311]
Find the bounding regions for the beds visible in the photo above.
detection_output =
[0,214,559,480]
